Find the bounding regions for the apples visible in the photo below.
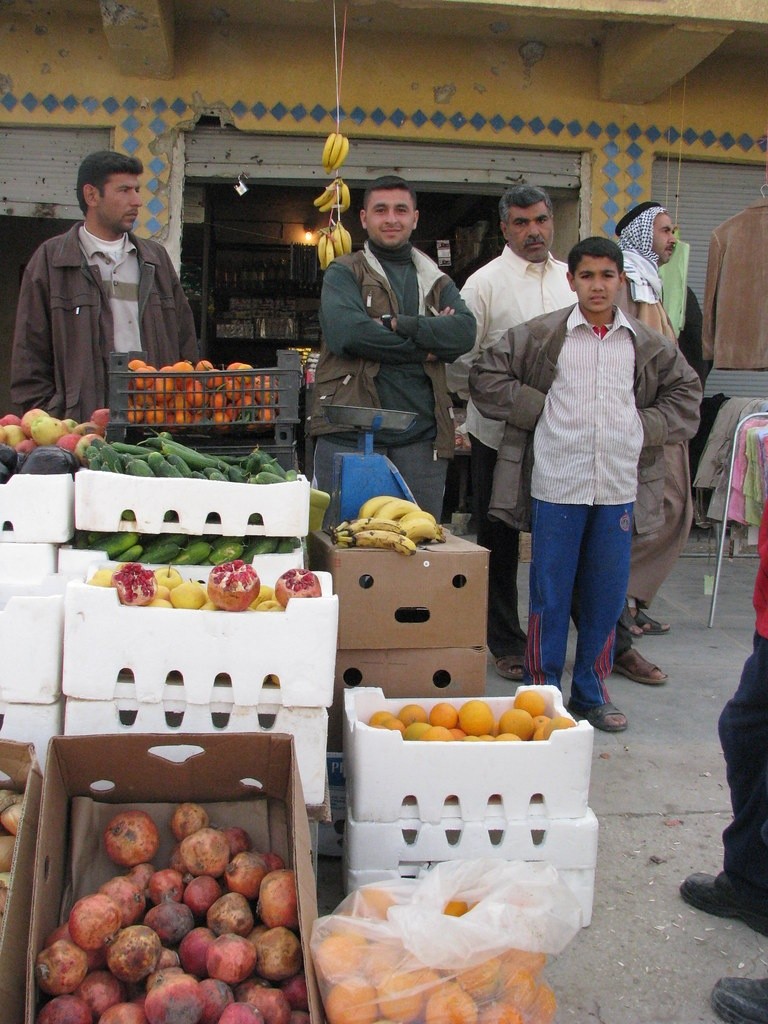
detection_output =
[0,407,111,466]
[86,567,285,611]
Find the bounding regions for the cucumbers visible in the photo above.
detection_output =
[74,432,301,565]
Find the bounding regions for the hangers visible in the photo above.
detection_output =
[760,183,768,197]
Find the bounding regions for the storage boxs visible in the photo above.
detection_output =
[57,539,308,587]
[311,525,492,651]
[316,753,345,859]
[339,864,597,929]
[106,423,301,473]
[72,467,311,539]
[0,571,68,706]
[340,685,597,825]
[61,556,340,709]
[0,473,76,543]
[0,736,44,1024]
[330,645,491,751]
[0,543,59,585]
[343,783,597,868]
[104,348,301,426]
[26,720,321,1024]
[60,697,332,810]
[0,696,66,771]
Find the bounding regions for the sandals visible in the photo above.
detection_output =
[612,649,669,684]
[568,697,628,733]
[493,654,525,679]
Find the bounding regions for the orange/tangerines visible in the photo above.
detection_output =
[369,690,575,740]
[313,885,556,1024]
[128,357,279,430]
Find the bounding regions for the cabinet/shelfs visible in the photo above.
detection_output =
[213,241,323,351]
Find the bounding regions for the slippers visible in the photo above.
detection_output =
[616,597,671,640]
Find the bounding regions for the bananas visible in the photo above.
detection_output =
[318,220,352,269]
[322,133,350,174]
[331,495,446,556]
[313,176,351,213]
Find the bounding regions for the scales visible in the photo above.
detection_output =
[322,403,435,536]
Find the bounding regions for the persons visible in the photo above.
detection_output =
[304,175,476,524]
[466,238,704,731]
[680,498,768,1024]
[8,150,200,425]
[446,180,671,687]
[615,202,694,639]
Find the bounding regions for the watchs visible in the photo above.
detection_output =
[379,312,395,331]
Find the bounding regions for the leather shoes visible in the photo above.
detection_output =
[679,873,768,938]
[710,976,768,1024]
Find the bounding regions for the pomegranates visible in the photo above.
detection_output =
[111,563,158,605]
[29,800,309,1024]
[207,560,260,610]
[276,569,322,607]
